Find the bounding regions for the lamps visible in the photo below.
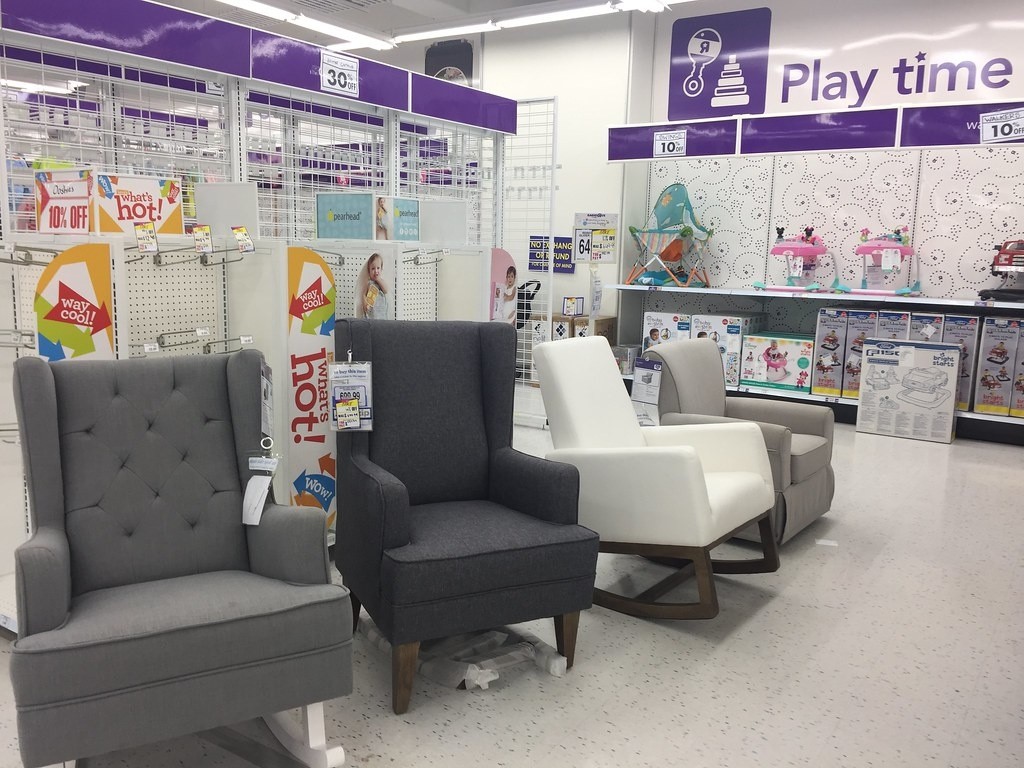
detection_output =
[222,0,692,52]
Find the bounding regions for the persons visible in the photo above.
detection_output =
[892,333,898,339]
[817,355,826,365]
[826,330,837,346]
[983,369,990,378]
[377,197,390,239]
[999,365,1006,372]
[646,328,661,347]
[697,332,708,339]
[1017,373,1024,380]
[959,338,968,353]
[496,265,516,326]
[858,331,867,340]
[747,351,753,359]
[359,253,389,322]
[768,341,787,360]
[996,342,1005,349]
[832,353,839,363]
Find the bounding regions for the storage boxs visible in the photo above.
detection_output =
[855,335,965,444]
[690,313,751,387]
[611,346,638,375]
[620,344,642,357]
[811,307,1024,418]
[739,334,815,394]
[528,312,617,388]
[641,311,695,353]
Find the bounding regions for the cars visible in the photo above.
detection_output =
[991,240,1024,277]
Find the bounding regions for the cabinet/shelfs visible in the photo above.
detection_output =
[603,282,1024,449]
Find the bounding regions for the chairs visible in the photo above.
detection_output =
[532,335,780,622]
[7,347,355,768]
[641,337,835,547]
[332,316,599,718]
